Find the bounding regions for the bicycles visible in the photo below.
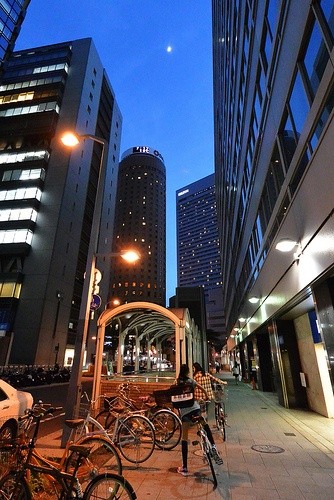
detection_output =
[213,383,230,441]
[0,378,183,500]
[189,398,218,488]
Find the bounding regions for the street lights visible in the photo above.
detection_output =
[61,131,142,449]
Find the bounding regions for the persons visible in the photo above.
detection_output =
[232,361,240,385]
[193,362,227,438]
[215,360,220,373]
[63,367,67,371]
[54,363,60,372]
[173,364,211,477]
[209,362,212,368]
[37,365,44,373]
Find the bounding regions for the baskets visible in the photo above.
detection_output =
[109,398,131,419]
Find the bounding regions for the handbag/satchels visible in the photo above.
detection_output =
[170,383,194,409]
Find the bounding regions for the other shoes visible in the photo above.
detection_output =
[211,445,223,465]
[177,467,188,476]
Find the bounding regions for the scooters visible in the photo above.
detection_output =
[0,366,72,387]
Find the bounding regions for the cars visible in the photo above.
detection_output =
[0,378,34,447]
